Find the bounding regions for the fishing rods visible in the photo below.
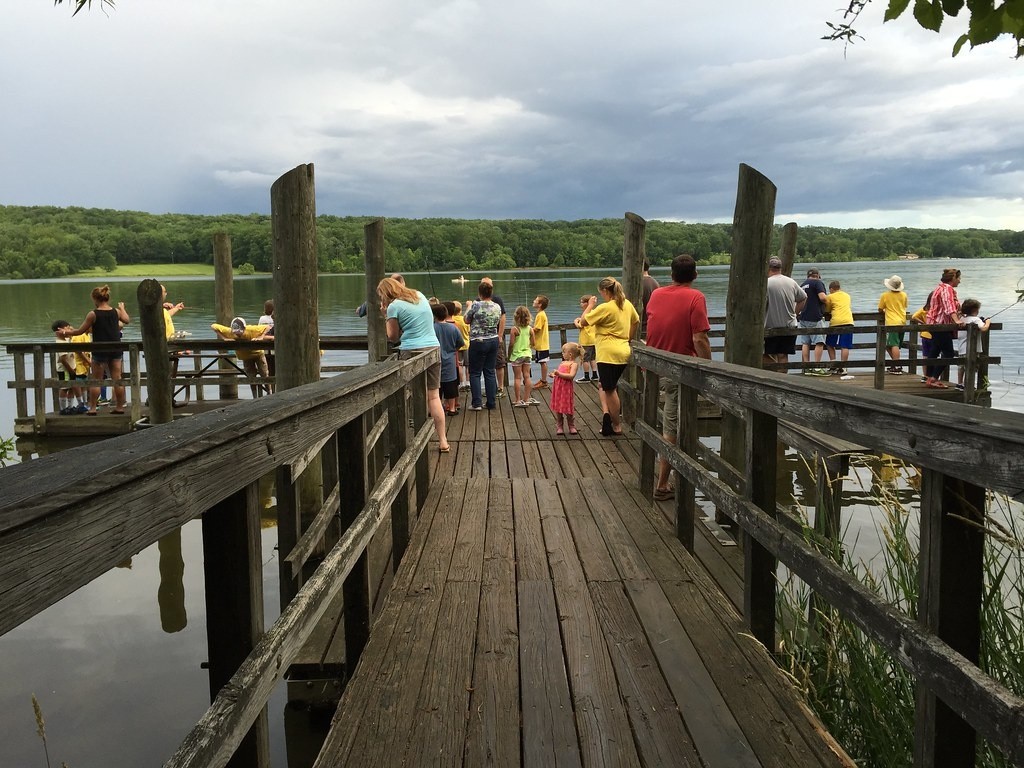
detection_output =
[521,276,527,308]
[421,240,435,296]
[509,263,520,305]
[983,300,1019,322]
[173,305,205,310]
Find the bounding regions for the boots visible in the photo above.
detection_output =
[567,416,577,434]
[557,417,564,434]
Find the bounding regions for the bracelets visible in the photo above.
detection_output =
[62,331,67,337]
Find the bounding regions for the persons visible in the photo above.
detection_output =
[53,319,129,414]
[879,268,994,391]
[161,285,185,406]
[581,275,642,436]
[356,272,450,452]
[642,258,661,338]
[763,258,809,378]
[53,284,129,415]
[210,319,272,399]
[645,254,712,498]
[507,306,540,408]
[824,282,854,374]
[259,298,275,377]
[531,295,551,390]
[548,342,586,435]
[461,277,508,411]
[429,297,469,416]
[798,267,832,378]
[573,295,599,384]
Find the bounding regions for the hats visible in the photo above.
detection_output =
[768,256,783,268]
[807,267,821,279]
[884,274,905,292]
[231,317,246,331]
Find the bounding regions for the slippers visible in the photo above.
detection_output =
[515,400,529,408]
[653,483,675,500]
[602,413,613,437]
[525,398,540,405]
[448,409,459,416]
[599,428,623,436]
[441,444,450,452]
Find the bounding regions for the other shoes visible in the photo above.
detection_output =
[468,405,482,411]
[455,403,461,409]
[446,404,450,410]
[482,404,488,409]
[803,365,976,392]
[495,387,507,398]
[575,377,590,384]
[482,390,486,397]
[590,377,598,381]
[60,397,128,416]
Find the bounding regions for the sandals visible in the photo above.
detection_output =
[533,379,549,389]
